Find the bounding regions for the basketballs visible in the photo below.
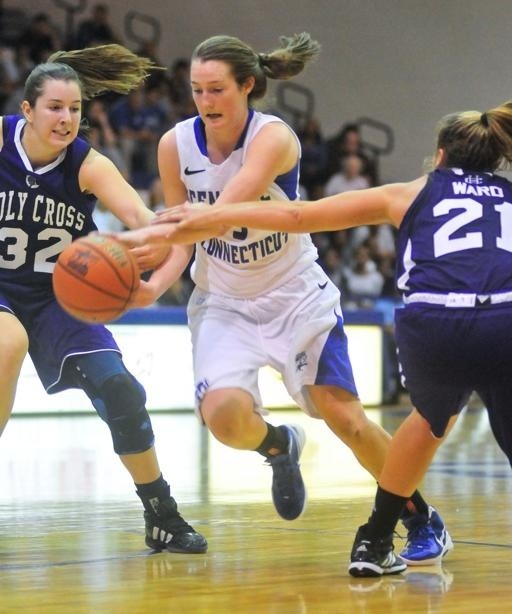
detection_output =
[53,236,140,324]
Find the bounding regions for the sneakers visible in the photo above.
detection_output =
[265,424,307,521]
[347,523,407,577]
[399,505,453,566]
[143,496,207,554]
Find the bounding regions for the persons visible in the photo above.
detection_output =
[152,98,512,579]
[1,38,208,558]
[90,29,455,565]
[0,0,398,306]
